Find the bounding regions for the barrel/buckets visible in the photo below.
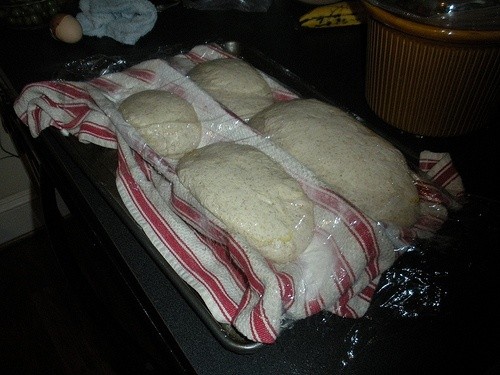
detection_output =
[363,2,500,145]
